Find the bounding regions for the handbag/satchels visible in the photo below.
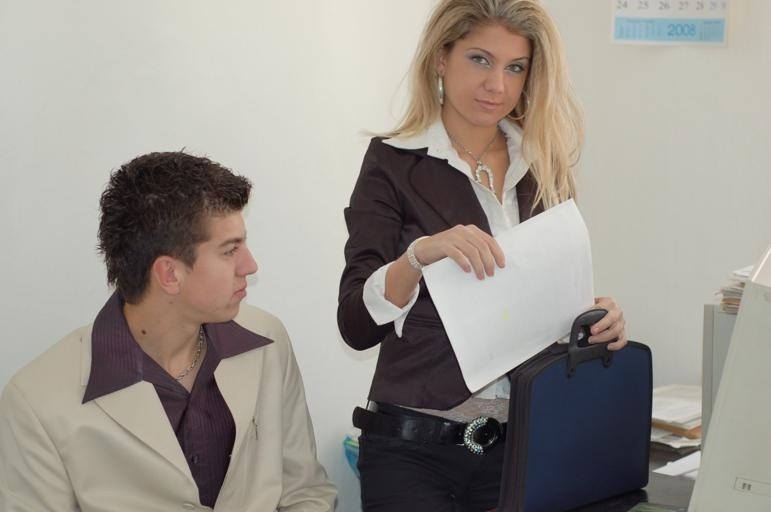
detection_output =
[500,309,654,512]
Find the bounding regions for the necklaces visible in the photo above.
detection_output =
[446,127,501,195]
[175,324,205,381]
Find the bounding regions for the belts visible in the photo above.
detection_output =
[351,401,503,457]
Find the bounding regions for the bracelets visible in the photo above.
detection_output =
[406,236,429,270]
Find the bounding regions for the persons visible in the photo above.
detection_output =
[0,151,338,512]
[337,1,628,512]
